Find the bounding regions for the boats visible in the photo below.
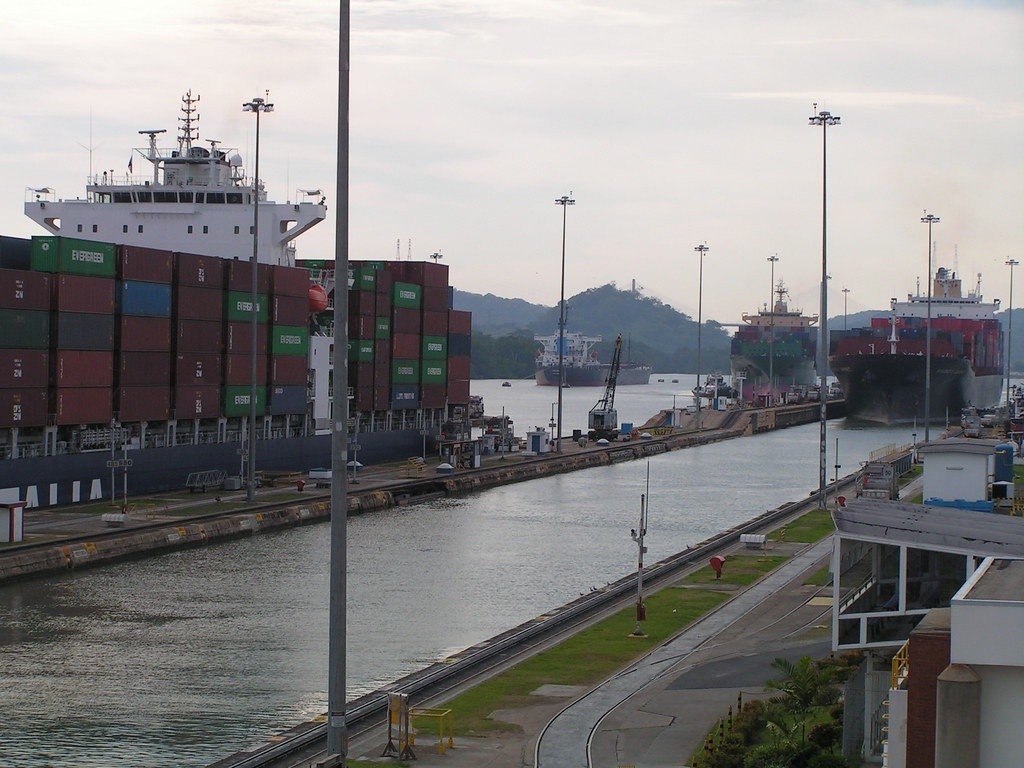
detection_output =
[534,300,651,386]
[691,371,736,399]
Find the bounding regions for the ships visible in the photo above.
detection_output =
[729,276,820,401]
[825,269,1007,425]
[2,87,512,514]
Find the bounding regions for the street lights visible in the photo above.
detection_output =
[765,254,780,409]
[1006,256,1018,432]
[239,94,274,505]
[919,213,940,442]
[554,195,575,454]
[694,243,710,431]
[808,107,841,512]
